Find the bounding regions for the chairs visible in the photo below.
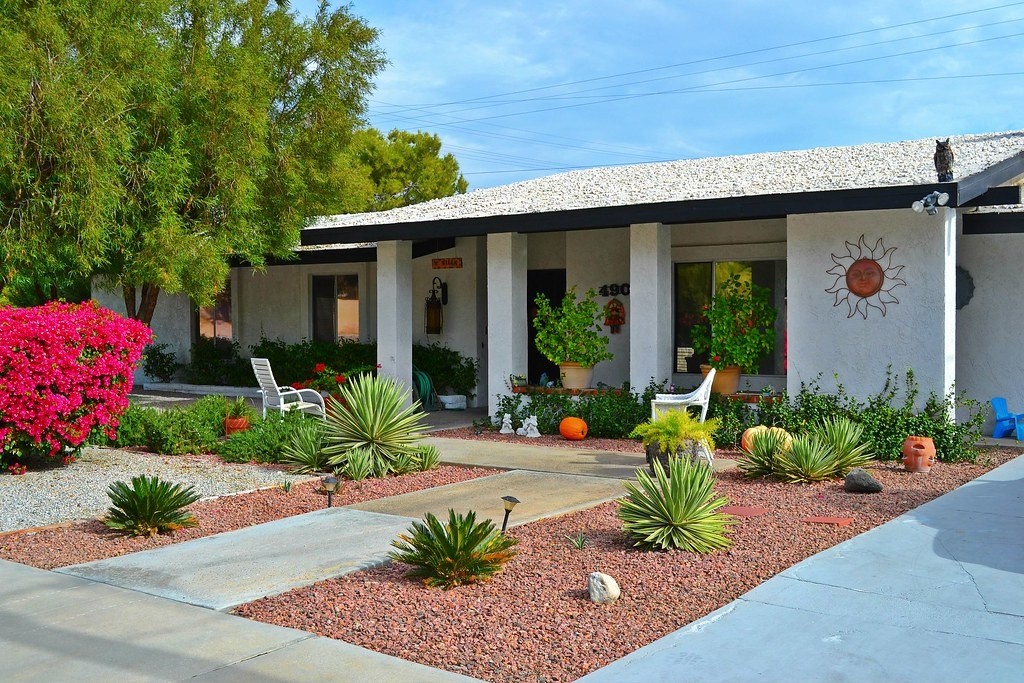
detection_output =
[651,369,717,466]
[991,396,1024,441]
[249,357,326,421]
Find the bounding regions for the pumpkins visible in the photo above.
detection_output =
[559,417,588,440]
[742,425,794,452]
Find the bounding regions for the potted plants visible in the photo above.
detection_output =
[224,396,250,437]
[628,409,722,479]
[534,285,614,389]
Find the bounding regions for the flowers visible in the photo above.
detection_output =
[691,272,778,402]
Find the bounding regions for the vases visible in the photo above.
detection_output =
[700,365,741,395]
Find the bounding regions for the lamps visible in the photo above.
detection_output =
[912,191,950,216]
[501,496,520,534]
[323,478,339,507]
[423,276,449,335]
